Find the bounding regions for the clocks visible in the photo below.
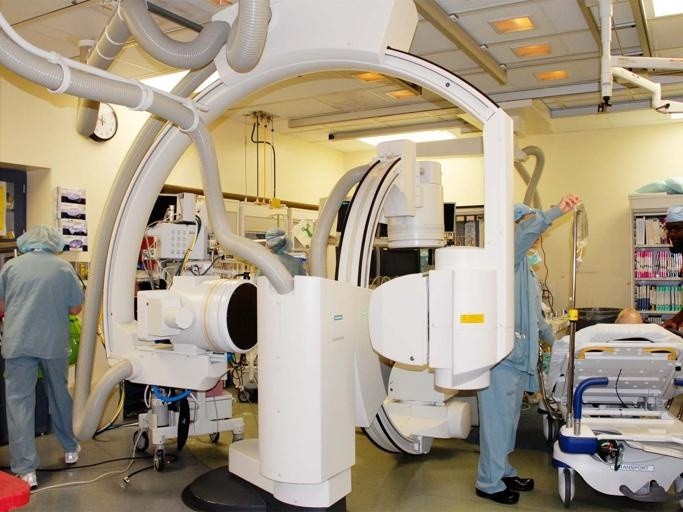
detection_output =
[88,102,119,143]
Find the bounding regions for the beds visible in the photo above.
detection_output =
[544,324,683,455]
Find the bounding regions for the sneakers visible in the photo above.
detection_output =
[63,441,83,466]
[474,484,520,506]
[16,470,40,492]
[500,475,535,493]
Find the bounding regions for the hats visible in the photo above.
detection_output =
[264,227,294,256]
[512,200,540,224]
[663,204,683,223]
[14,223,68,256]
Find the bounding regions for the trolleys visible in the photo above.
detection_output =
[133,387,244,473]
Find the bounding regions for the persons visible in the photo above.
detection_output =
[658,207,683,332]
[615,308,644,323]
[0,226,85,490]
[475,193,581,504]
[256,227,307,286]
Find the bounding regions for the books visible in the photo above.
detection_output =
[635,284,656,311]
[656,285,683,311]
[635,250,654,278]
[659,251,682,278]
[649,316,663,326]
[636,218,668,245]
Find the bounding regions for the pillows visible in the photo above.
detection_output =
[634,181,668,192]
[663,177,683,192]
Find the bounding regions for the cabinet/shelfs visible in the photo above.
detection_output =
[629,194,682,333]
